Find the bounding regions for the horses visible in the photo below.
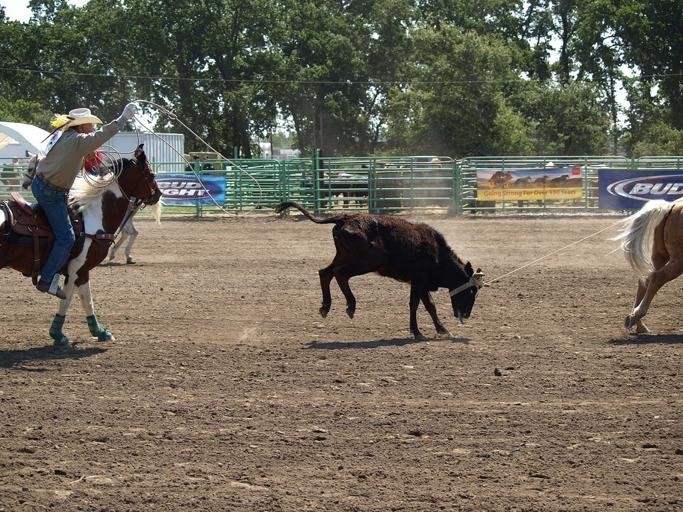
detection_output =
[22,149,162,264]
[0,143,163,354]
[605,196,683,335]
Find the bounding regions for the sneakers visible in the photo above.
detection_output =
[35,278,66,301]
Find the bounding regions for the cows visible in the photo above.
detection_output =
[272,200,485,341]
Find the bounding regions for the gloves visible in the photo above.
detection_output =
[122,102,142,120]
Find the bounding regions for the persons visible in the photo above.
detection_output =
[30,102,142,299]
[0,157,22,186]
[83,147,102,173]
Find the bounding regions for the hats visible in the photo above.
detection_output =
[63,107,104,132]
